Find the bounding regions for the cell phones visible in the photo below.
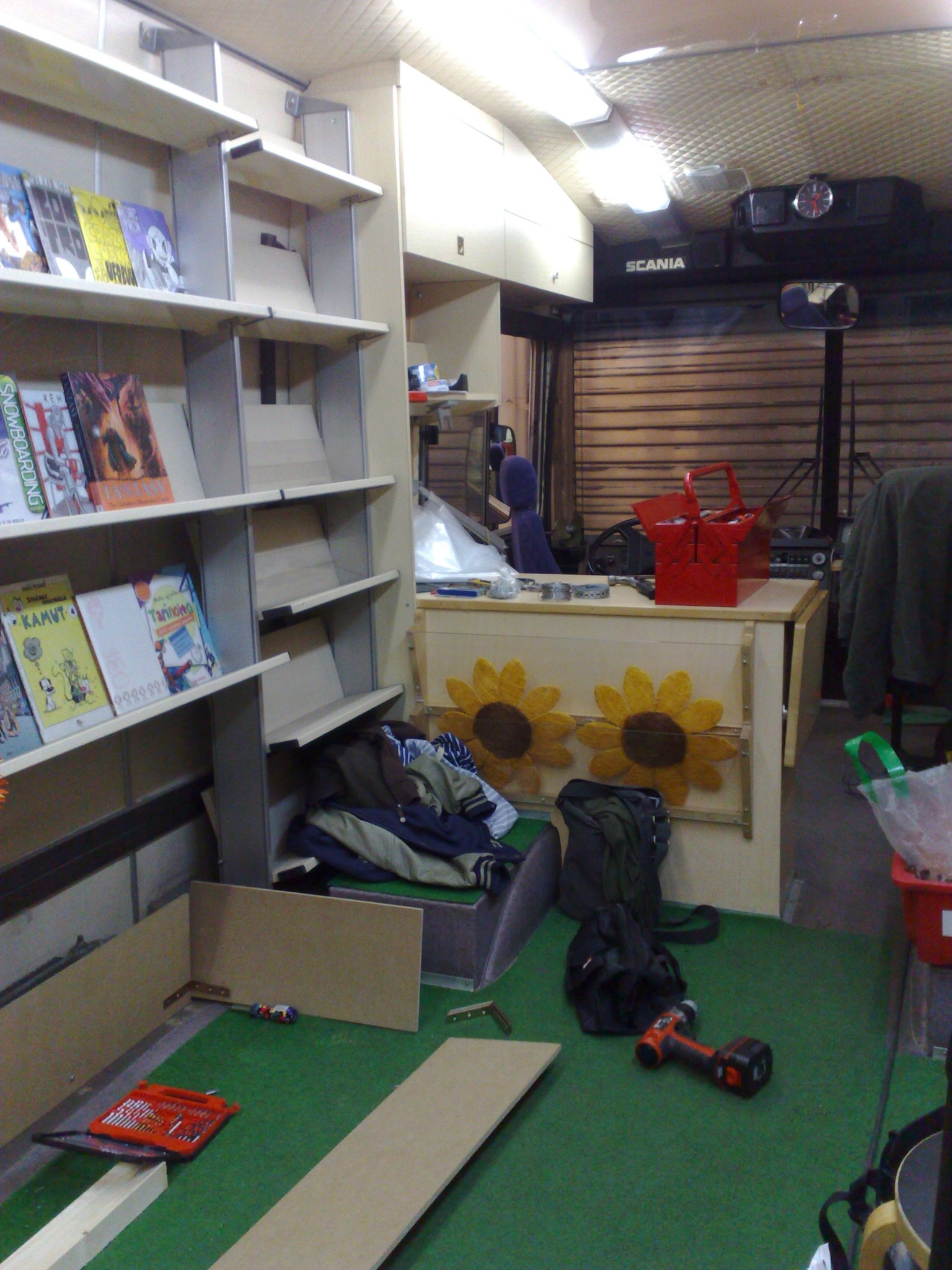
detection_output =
[515,578,534,585]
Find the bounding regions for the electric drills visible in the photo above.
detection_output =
[637,999,773,1098]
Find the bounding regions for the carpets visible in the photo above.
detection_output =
[0,818,952,1270]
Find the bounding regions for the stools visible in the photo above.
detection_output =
[859,1130,944,1270]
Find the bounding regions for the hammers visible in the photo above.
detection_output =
[608,575,655,600]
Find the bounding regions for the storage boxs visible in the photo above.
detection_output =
[890,851,952,966]
[631,461,795,607]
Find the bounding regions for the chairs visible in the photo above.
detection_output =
[780,287,832,327]
[500,455,563,575]
[587,516,641,575]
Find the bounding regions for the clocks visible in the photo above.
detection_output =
[793,179,833,220]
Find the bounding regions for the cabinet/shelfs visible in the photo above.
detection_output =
[0,0,836,1002]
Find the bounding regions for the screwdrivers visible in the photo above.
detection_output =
[224,1003,297,1024]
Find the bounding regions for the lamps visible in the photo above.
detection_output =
[399,0,693,250]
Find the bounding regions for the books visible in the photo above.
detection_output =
[0,369,175,524]
[0,163,187,294]
[0,561,223,760]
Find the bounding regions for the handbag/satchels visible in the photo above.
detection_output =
[556,778,670,925]
[844,732,952,881]
[563,905,689,1037]
